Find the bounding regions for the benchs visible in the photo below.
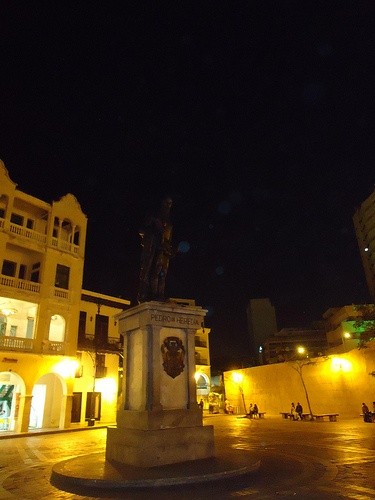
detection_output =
[279,412,311,420]
[360,412,375,423]
[246,412,266,419]
[315,412,339,421]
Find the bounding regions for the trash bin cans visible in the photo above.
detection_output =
[86,417,96,426]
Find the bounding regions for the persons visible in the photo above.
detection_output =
[290,401,304,421]
[248,403,258,419]
[137,195,174,299]
[198,399,203,408]
[362,402,375,421]
